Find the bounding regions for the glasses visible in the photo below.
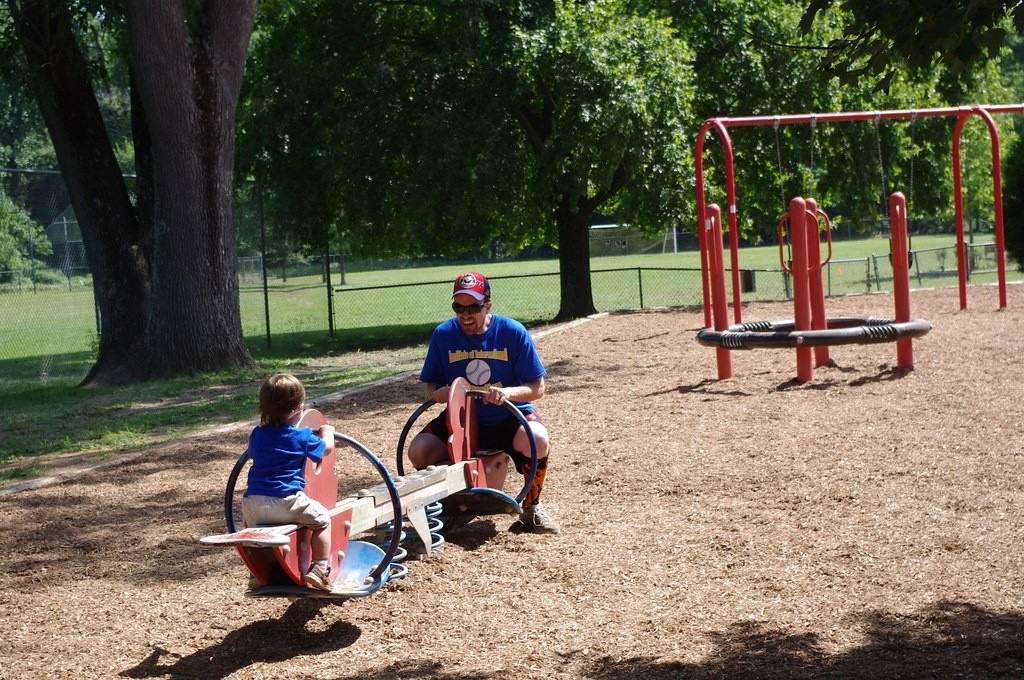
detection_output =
[452,302,487,314]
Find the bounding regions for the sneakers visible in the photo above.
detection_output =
[519,504,560,534]
[303,561,333,591]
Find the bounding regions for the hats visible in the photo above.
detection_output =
[451,272,491,303]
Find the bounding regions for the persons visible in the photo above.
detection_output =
[243,374,335,594]
[408,273,558,535]
